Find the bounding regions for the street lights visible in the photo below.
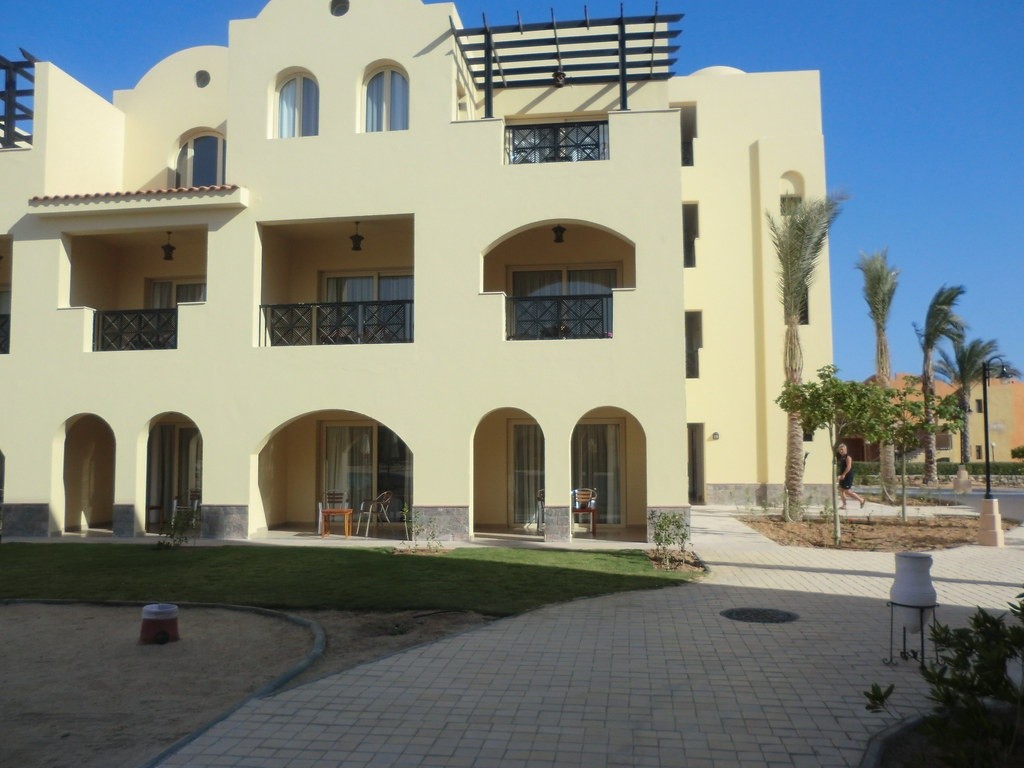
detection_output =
[980,355,1013,499]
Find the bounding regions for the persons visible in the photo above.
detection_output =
[835,444,865,509]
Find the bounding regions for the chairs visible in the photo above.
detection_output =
[356,491,393,537]
[537,488,545,532]
[568,487,597,535]
[317,489,348,534]
[168,493,202,533]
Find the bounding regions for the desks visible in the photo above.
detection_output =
[321,509,353,538]
[145,506,164,535]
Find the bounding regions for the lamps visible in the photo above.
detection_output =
[713,432,719,439]
[163,231,176,260]
[552,224,565,243]
[552,61,565,86]
[349,221,363,251]
